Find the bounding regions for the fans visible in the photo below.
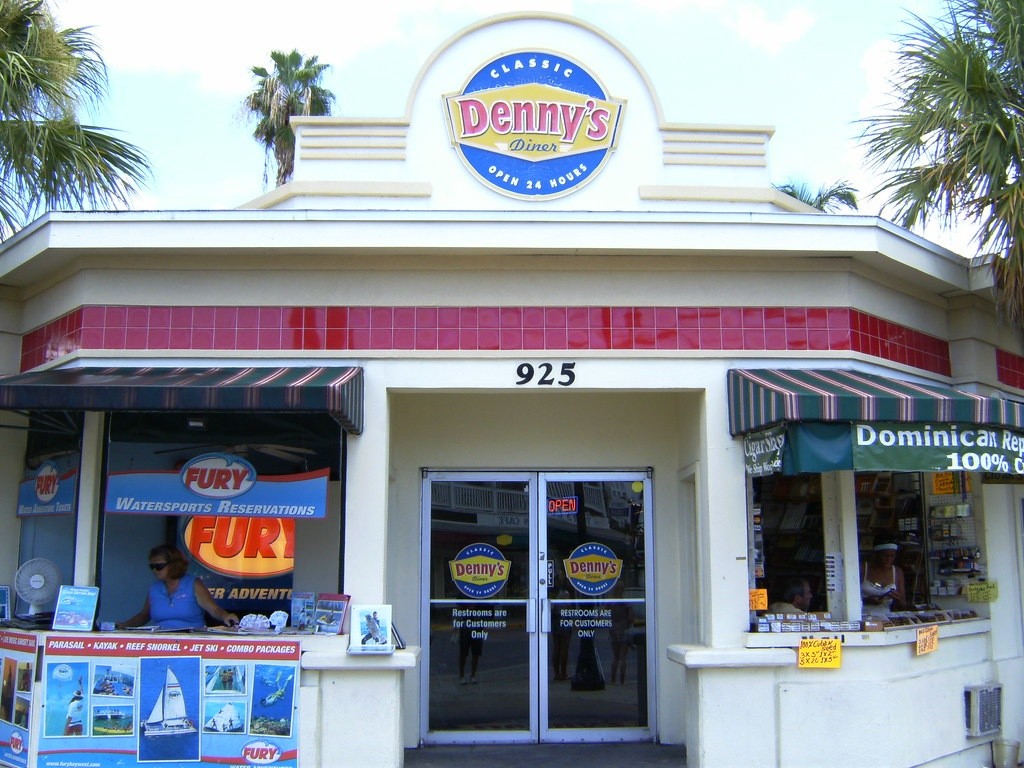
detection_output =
[16,557,63,618]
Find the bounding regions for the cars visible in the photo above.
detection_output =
[624,587,646,647]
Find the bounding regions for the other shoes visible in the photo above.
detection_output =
[620,673,625,684]
[459,677,467,685]
[611,670,617,682]
[469,675,477,683]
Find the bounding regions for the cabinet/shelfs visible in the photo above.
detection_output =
[891,472,930,604]
[927,516,981,577]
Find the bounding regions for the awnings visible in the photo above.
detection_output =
[0,368,364,434]
[727,369,1024,475]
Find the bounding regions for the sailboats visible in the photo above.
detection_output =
[145,666,197,739]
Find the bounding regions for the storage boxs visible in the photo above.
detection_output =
[929,587,938,595]
[938,586,947,596]
[947,579,960,587]
[947,586,962,595]
[934,579,944,587]
[753,608,983,633]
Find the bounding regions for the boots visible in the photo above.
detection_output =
[553,664,563,681]
[562,662,571,680]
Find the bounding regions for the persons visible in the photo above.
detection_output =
[859,535,906,613]
[456,580,634,687]
[361,611,387,644]
[768,578,813,612]
[115,544,238,629]
[4,660,293,736]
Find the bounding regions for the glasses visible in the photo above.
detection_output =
[149,563,169,572]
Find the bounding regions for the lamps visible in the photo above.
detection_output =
[184,413,209,432]
